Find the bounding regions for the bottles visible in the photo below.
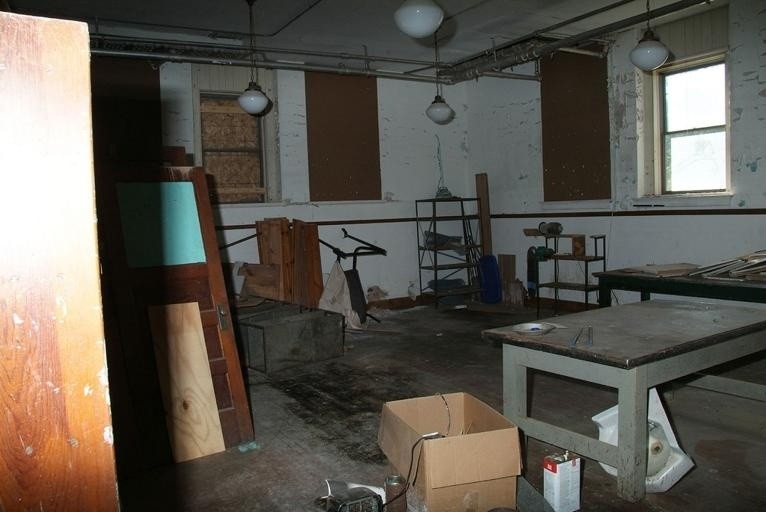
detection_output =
[385,475,408,512]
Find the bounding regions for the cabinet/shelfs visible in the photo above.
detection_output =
[416,197,484,311]
[534,233,606,319]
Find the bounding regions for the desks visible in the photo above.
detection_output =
[481,297,765,503]
[595,263,766,401]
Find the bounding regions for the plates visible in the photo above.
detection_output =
[511,322,556,336]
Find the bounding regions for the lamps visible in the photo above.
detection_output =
[236,1,269,115]
[394,1,452,123]
[630,1,670,72]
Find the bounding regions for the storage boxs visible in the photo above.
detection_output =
[378,392,525,511]
